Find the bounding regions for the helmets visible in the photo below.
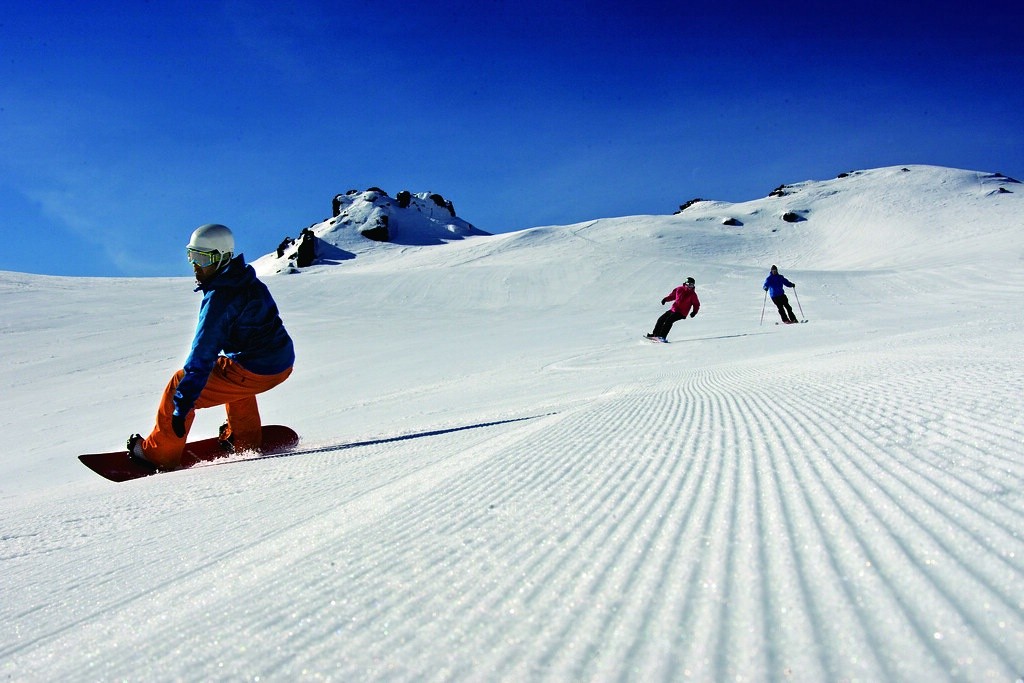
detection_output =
[187,224,235,269]
[685,277,695,285]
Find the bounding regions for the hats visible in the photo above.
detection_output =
[771,265,777,270]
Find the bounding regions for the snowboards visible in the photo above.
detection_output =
[643,334,669,344]
[78,423,299,483]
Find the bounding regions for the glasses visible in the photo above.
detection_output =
[189,248,230,267]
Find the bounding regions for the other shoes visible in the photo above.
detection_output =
[125,434,148,466]
[785,319,798,324]
[219,425,234,446]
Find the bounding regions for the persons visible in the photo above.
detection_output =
[647,277,700,340]
[763,265,798,324]
[126,224,295,471]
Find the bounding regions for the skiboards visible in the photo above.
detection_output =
[774,319,808,323]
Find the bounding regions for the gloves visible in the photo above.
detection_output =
[662,297,667,305]
[690,312,696,317]
[171,416,186,437]
